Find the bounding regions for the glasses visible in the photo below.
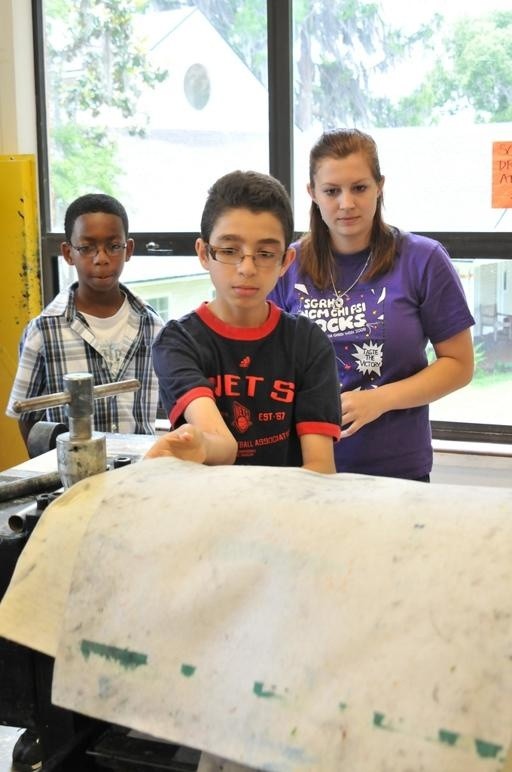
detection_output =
[68,241,127,257]
[204,245,286,268]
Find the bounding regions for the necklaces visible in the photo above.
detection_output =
[329,252,370,308]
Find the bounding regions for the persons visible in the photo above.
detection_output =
[266,128,475,482]
[142,170,342,475]
[5,195,164,459]
